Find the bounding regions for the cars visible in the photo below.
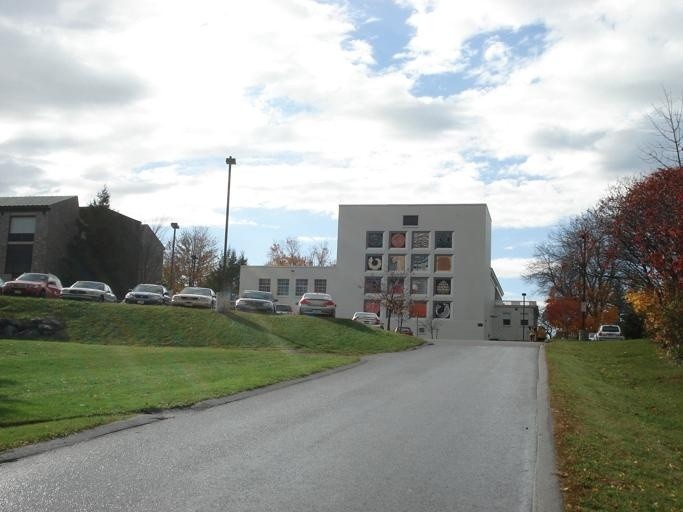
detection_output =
[594,325,624,341]
[2,273,63,297]
[123,282,171,305]
[296,292,337,318]
[60,280,119,304]
[587,332,596,341]
[353,311,381,327]
[395,326,414,336]
[235,289,278,313]
[275,303,296,315]
[171,286,218,310]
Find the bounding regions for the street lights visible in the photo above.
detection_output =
[521,292,527,343]
[580,232,591,328]
[189,254,198,285]
[169,223,180,289]
[220,155,237,290]
